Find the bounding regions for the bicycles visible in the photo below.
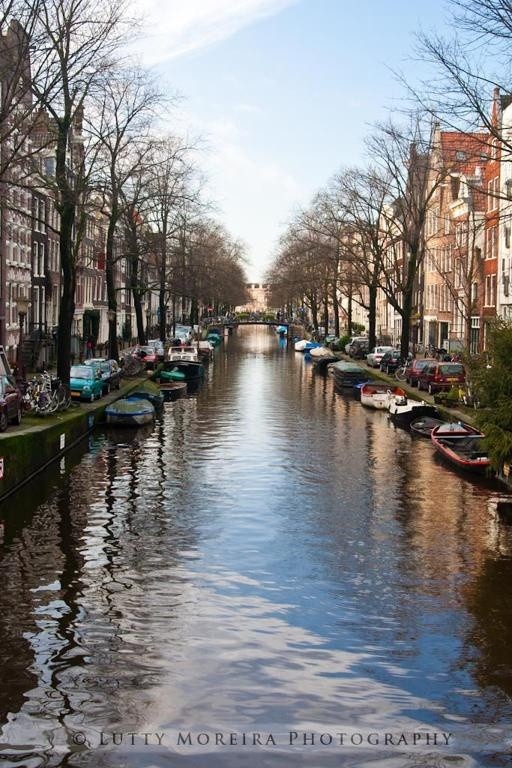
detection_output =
[23,370,72,416]
[394,355,414,382]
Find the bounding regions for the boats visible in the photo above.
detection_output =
[351,378,497,471]
[105,329,222,427]
[291,337,369,387]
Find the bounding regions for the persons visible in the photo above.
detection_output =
[86,334,97,359]
[226,310,230,317]
[277,309,282,323]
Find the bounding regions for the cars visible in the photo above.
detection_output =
[405,358,466,395]
[312,327,345,350]
[0,375,23,432]
[169,324,202,346]
[70,358,121,402]
[344,336,413,374]
